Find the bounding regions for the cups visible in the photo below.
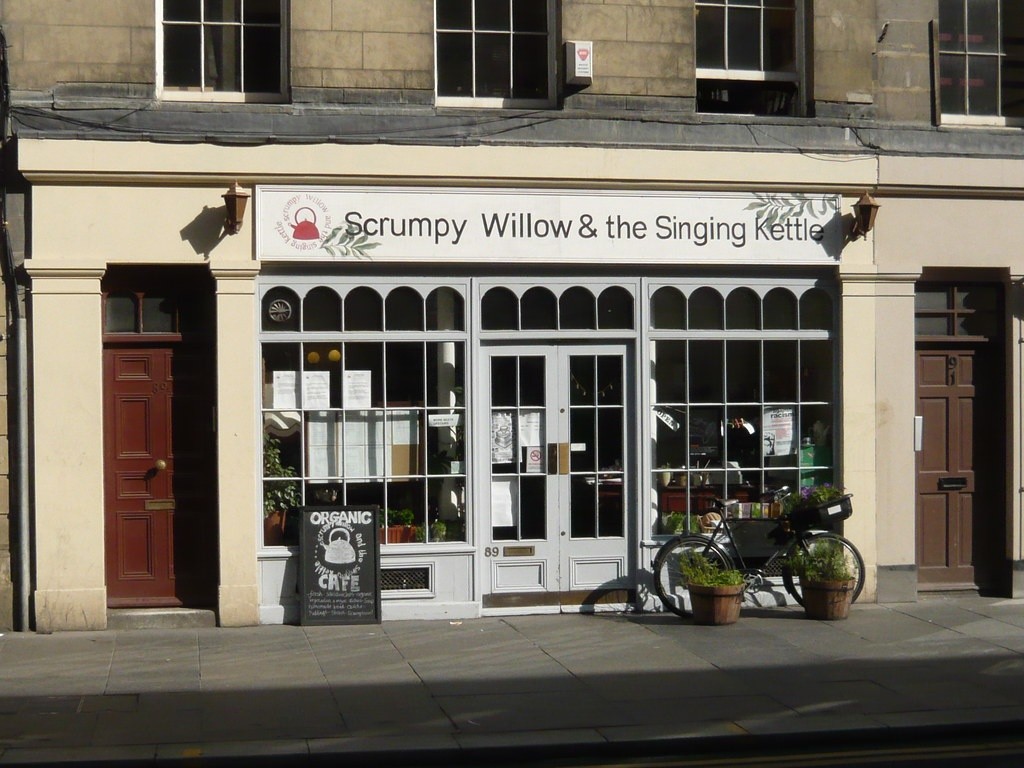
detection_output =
[659,472,670,487]
[694,473,703,486]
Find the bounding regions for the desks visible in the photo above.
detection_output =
[595,483,622,502]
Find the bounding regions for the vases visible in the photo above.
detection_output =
[785,494,853,525]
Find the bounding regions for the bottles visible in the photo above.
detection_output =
[680,466,687,487]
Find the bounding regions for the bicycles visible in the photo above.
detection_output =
[653,485,866,619]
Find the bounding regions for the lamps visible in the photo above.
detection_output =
[850,192,882,241]
[222,179,252,235]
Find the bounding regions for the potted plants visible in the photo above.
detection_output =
[263,430,302,545]
[679,545,747,625]
[782,535,859,620]
[379,508,446,542]
[659,462,670,487]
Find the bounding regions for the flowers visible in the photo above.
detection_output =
[782,482,845,513]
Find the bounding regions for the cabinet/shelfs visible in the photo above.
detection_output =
[660,485,755,514]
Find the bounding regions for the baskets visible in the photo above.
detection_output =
[811,493,854,525]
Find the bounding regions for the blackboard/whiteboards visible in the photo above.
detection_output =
[299,504,381,628]
